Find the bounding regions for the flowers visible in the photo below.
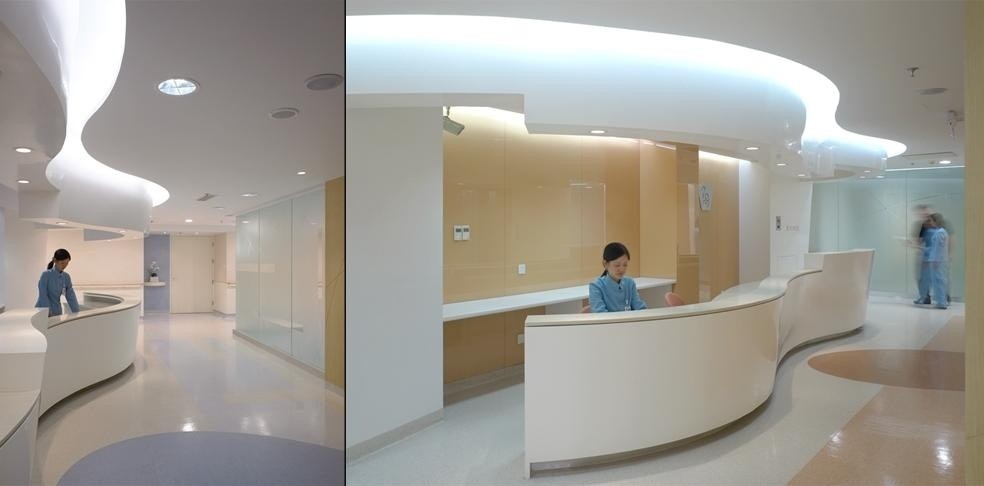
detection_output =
[148,260,160,277]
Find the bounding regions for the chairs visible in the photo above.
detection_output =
[665,291,685,306]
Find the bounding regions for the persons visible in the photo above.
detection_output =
[587,241,648,313]
[905,201,952,307]
[33,248,81,319]
[911,211,952,310]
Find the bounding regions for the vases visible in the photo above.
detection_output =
[149,276,159,282]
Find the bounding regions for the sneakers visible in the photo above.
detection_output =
[914,295,950,309]
